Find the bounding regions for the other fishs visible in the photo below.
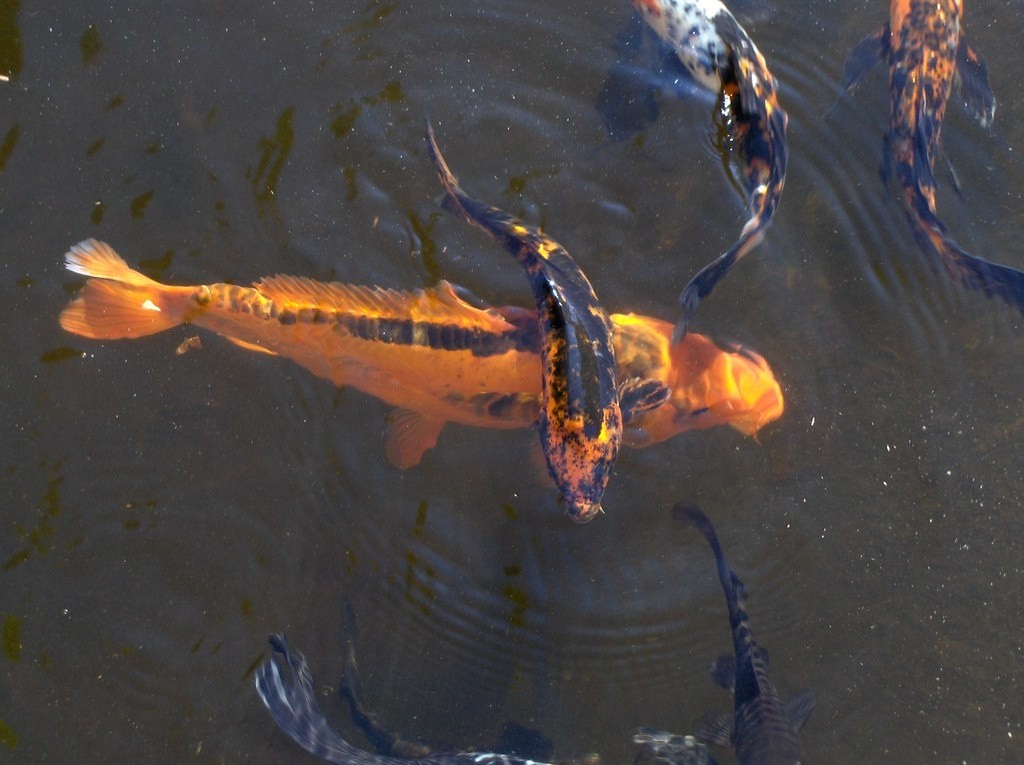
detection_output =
[822,0,1024,312]
[627,1,790,346]
[54,113,783,526]
[251,503,816,765]
